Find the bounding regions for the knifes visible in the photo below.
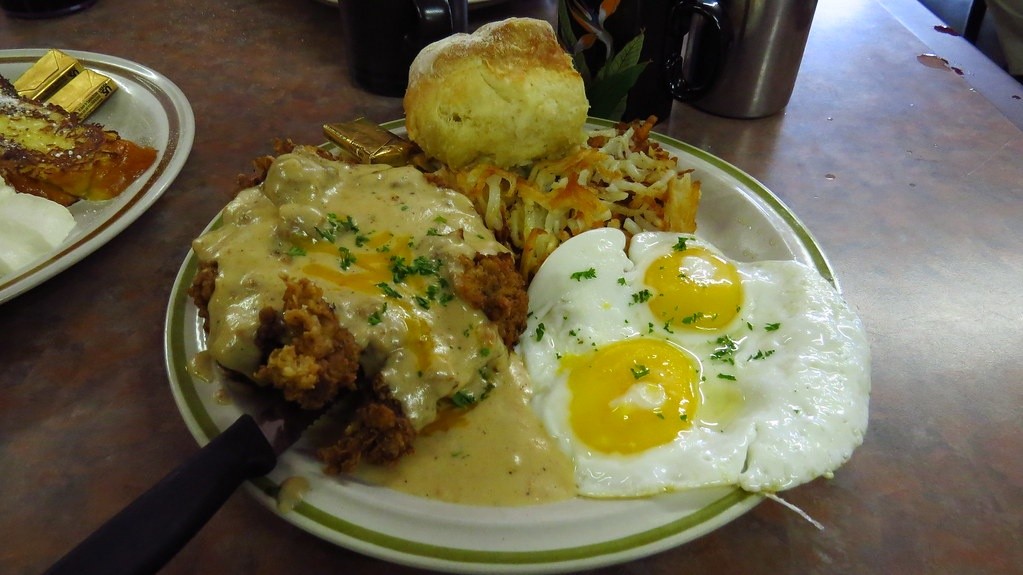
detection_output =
[39,342,387,575]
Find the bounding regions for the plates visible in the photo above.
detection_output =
[164,117,842,575]
[0,48,195,304]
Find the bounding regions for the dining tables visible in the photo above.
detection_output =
[0,0,1023,575]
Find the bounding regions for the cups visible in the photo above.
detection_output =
[338,0,468,98]
[557,0,732,121]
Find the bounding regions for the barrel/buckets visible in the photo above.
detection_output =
[673,0,818,120]
